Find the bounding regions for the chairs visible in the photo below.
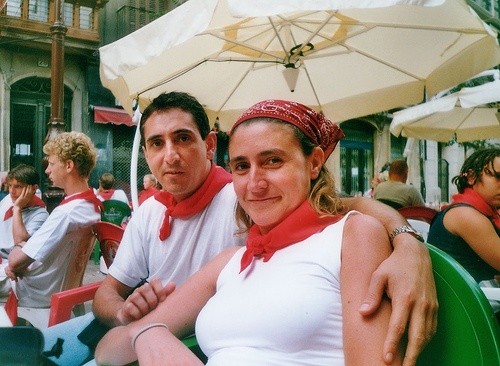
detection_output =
[1,204,500,366]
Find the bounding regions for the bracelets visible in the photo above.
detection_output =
[131,324,169,349]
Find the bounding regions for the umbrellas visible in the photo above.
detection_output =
[99,0,500,134]
[390,79,500,144]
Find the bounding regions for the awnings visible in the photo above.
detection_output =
[90,105,136,126]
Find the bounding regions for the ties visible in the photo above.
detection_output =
[57,187,105,213]
[4,195,45,220]
[154,161,232,241]
[452,187,500,229]
[240,199,343,274]
[100,189,116,201]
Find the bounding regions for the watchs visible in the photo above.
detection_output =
[390,225,425,243]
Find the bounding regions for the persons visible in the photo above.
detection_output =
[427,148,500,288]
[139,173,160,206]
[0,165,49,257]
[0,132,104,306]
[96,174,128,203]
[95,100,402,366]
[374,161,423,206]
[91,91,439,366]
